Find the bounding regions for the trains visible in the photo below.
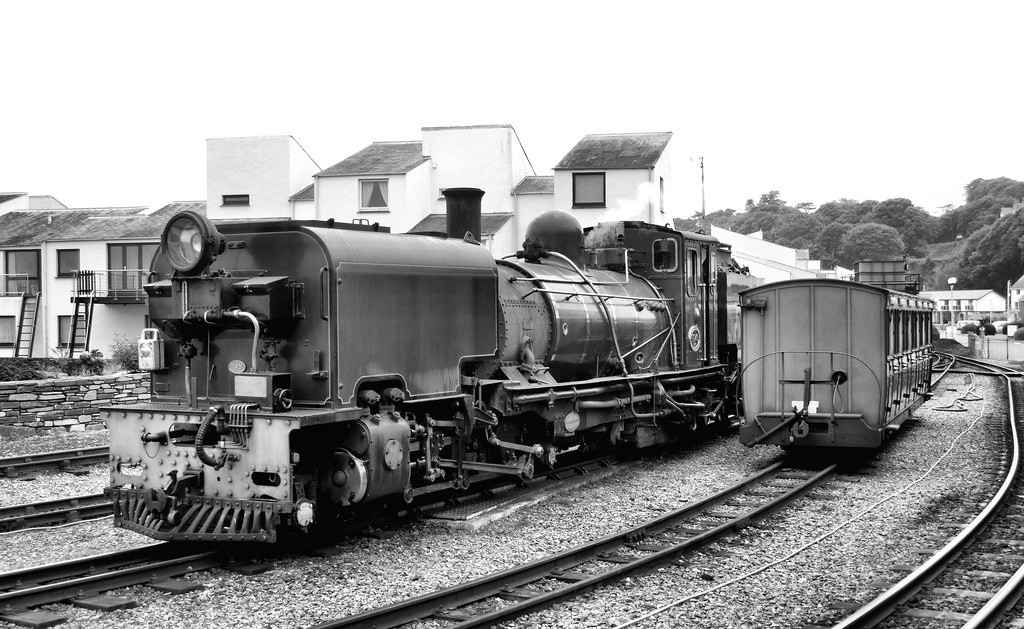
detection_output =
[736,253,935,448]
[99,187,766,544]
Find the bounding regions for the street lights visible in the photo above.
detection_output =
[948,277,956,339]
